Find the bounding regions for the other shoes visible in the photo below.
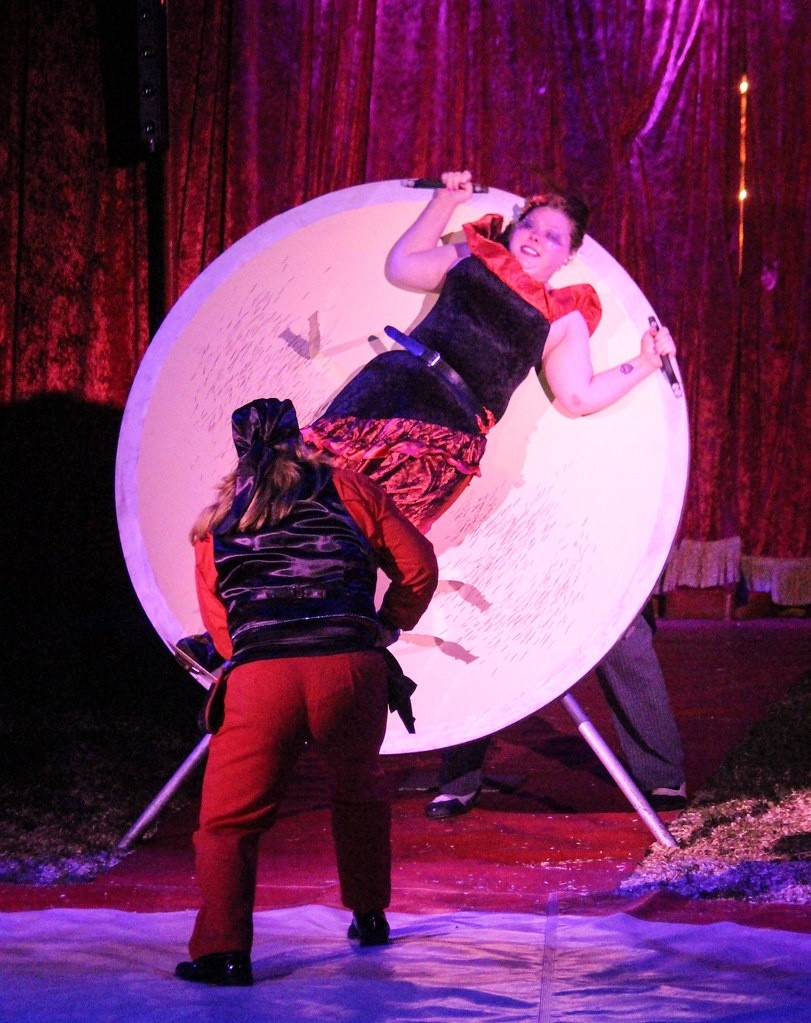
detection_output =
[179,629,223,671]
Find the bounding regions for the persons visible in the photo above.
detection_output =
[425,609,689,820]
[171,167,676,678]
[175,399,439,987]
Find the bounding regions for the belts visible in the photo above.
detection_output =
[385,325,490,427]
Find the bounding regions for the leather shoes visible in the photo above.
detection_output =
[349,910,389,948]
[176,951,251,987]
[428,789,481,818]
[649,779,687,811]
